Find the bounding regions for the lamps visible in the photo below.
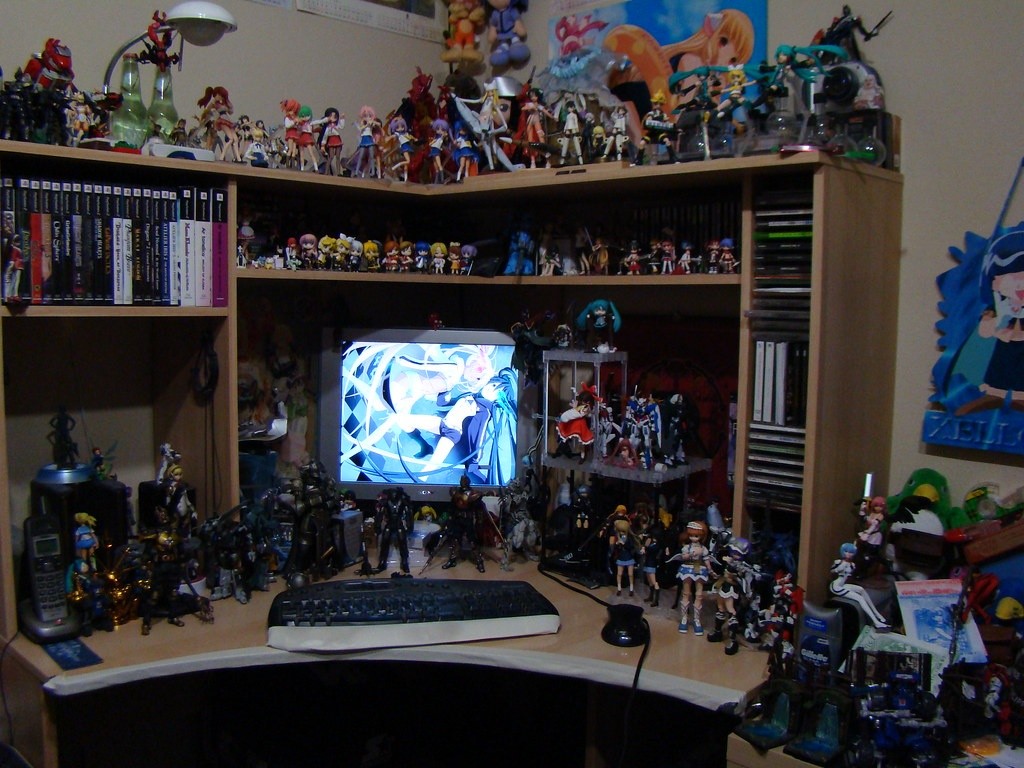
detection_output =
[102,3,239,98]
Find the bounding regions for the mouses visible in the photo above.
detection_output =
[601,604,649,647]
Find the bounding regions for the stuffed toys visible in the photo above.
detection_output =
[486,76,525,130]
[440,0,530,67]
[883,467,975,537]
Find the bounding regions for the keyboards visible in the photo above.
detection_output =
[266,577,559,653]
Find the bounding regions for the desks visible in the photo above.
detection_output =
[1,550,776,768]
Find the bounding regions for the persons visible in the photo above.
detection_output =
[46,398,542,641]
[240,188,739,275]
[354,80,508,183]
[557,484,803,669]
[144,10,179,71]
[829,497,899,631]
[630,5,892,166]
[521,88,628,169]
[168,86,347,177]
[6,234,23,303]
[0,69,93,149]
[553,300,733,472]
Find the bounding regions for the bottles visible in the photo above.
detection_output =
[111,53,145,142]
[149,60,177,136]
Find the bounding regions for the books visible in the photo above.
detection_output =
[0,178,229,308]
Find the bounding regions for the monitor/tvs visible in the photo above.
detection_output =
[317,327,537,502]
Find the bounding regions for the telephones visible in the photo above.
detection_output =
[19,495,86,645]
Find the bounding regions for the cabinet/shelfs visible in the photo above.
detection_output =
[0,138,904,649]
